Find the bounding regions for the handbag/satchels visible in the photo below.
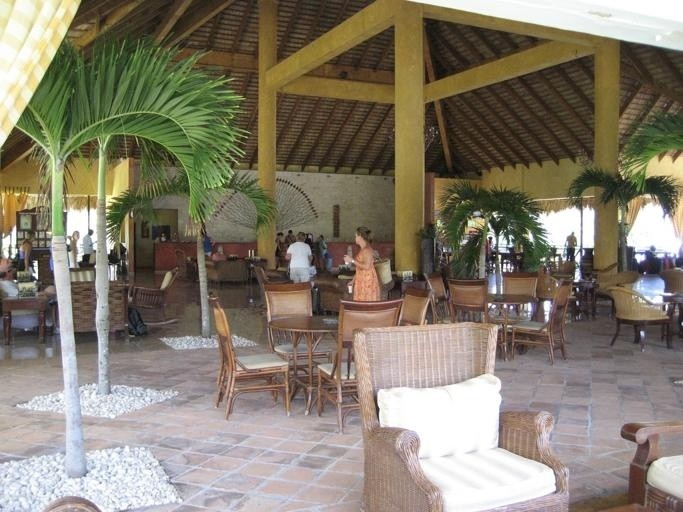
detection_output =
[373,259,396,293]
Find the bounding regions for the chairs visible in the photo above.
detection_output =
[424,250,683,364]
[176,249,266,289]
[354,323,571,511]
[1,243,179,343]
[207,265,432,433]
[621,421,683,512]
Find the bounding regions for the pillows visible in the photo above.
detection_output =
[378,372,501,455]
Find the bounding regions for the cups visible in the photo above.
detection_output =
[345,261,349,264]
[348,286,353,294]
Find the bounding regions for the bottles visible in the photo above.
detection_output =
[347,245,352,259]
[160,233,167,242]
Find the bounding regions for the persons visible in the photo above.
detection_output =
[285,231,314,282]
[66,231,81,268]
[212,243,227,261]
[637,240,683,275]
[17,230,37,275]
[562,230,577,261]
[202,230,217,257]
[342,225,381,303]
[308,255,317,278]
[82,228,97,266]
[486,236,496,269]
[275,230,328,271]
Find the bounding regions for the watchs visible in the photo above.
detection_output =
[351,258,355,264]
